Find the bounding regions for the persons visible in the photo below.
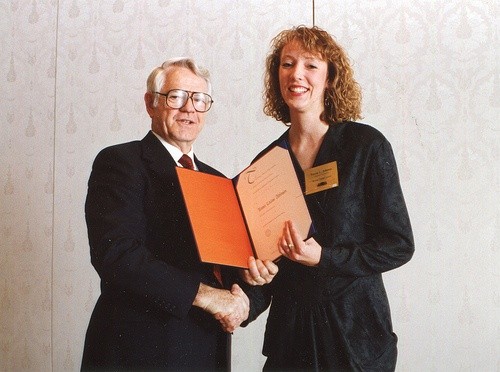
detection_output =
[81,57,279,372]
[214,25,415,372]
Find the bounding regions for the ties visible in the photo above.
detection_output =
[178,154,194,171]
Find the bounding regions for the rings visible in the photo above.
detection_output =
[255,276,261,280]
[289,244,294,247]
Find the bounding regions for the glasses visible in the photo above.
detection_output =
[155,89,214,112]
